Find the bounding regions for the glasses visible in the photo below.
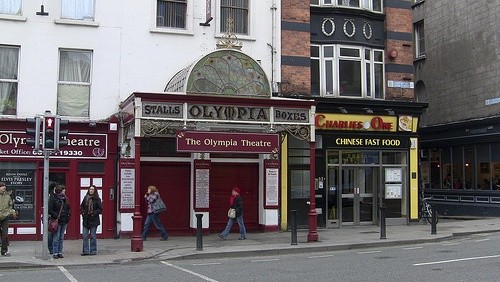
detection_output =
[1,185,6,187]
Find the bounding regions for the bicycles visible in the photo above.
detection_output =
[419,192,439,224]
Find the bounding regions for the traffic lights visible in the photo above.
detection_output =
[25,115,69,153]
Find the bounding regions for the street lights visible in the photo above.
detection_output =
[307,105,319,243]
[130,97,144,252]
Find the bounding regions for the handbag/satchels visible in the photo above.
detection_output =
[151,192,167,214]
[227,208,236,218]
[48,217,60,232]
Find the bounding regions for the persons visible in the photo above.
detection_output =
[141,185,169,242]
[41,183,59,254]
[217,187,248,241]
[80,185,102,256]
[0,182,19,257]
[48,185,72,259]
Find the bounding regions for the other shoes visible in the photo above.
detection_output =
[238,237,246,240]
[58,254,64,258]
[3,252,11,256]
[50,251,53,254]
[217,233,226,241]
[53,254,58,259]
[160,236,168,241]
[81,252,97,257]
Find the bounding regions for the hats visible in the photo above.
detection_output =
[0,182,6,187]
[232,187,240,195]
